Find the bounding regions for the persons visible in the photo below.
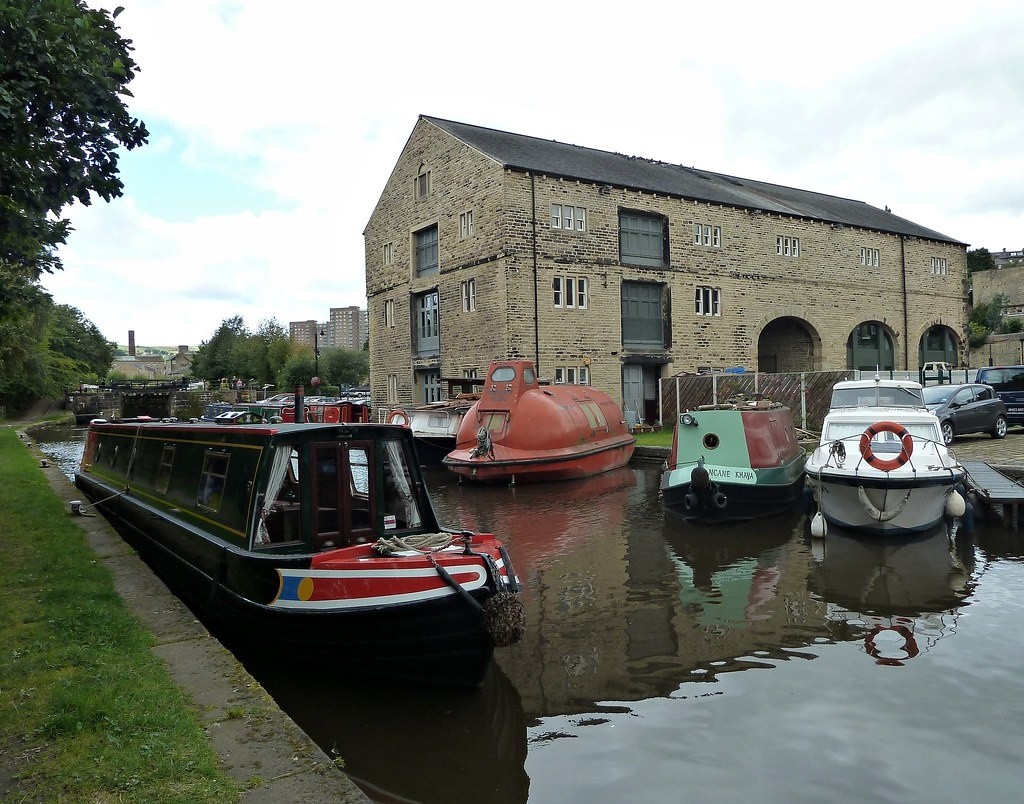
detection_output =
[233,376,242,389]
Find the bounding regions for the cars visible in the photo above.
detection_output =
[920,383,1009,445]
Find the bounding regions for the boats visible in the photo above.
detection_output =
[439,359,637,486]
[383,394,482,463]
[658,505,802,637]
[807,523,981,667]
[801,366,967,536]
[658,390,810,524]
[203,393,372,426]
[74,415,528,708]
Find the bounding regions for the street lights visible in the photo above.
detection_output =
[315,328,325,377]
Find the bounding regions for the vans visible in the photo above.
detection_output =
[974,365,1024,428]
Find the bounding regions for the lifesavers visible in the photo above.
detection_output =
[712,493,727,508]
[860,420,914,472]
[388,410,408,425]
[685,494,697,510]
[284,393,295,396]
